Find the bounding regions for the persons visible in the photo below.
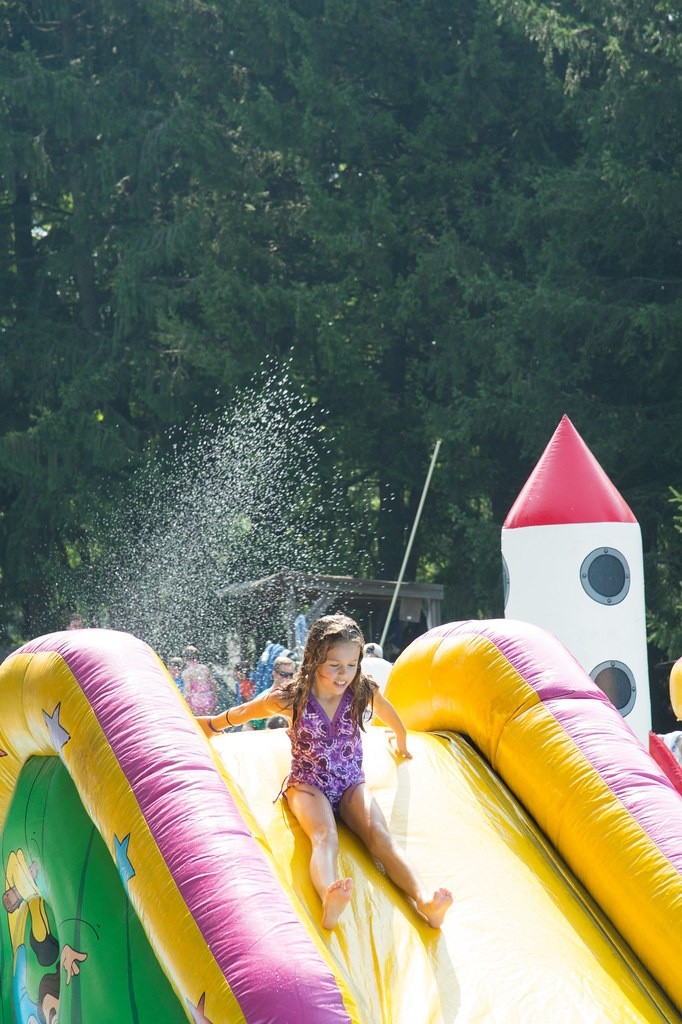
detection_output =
[192,615,454,931]
[362,642,383,659]
[167,645,306,733]
[66,612,83,630]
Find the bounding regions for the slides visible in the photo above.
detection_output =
[0,615,682,1023]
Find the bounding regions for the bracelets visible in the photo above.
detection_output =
[226,708,239,727]
[208,717,225,733]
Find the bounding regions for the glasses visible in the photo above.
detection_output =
[277,670,293,679]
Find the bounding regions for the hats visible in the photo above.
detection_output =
[362,643,383,659]
